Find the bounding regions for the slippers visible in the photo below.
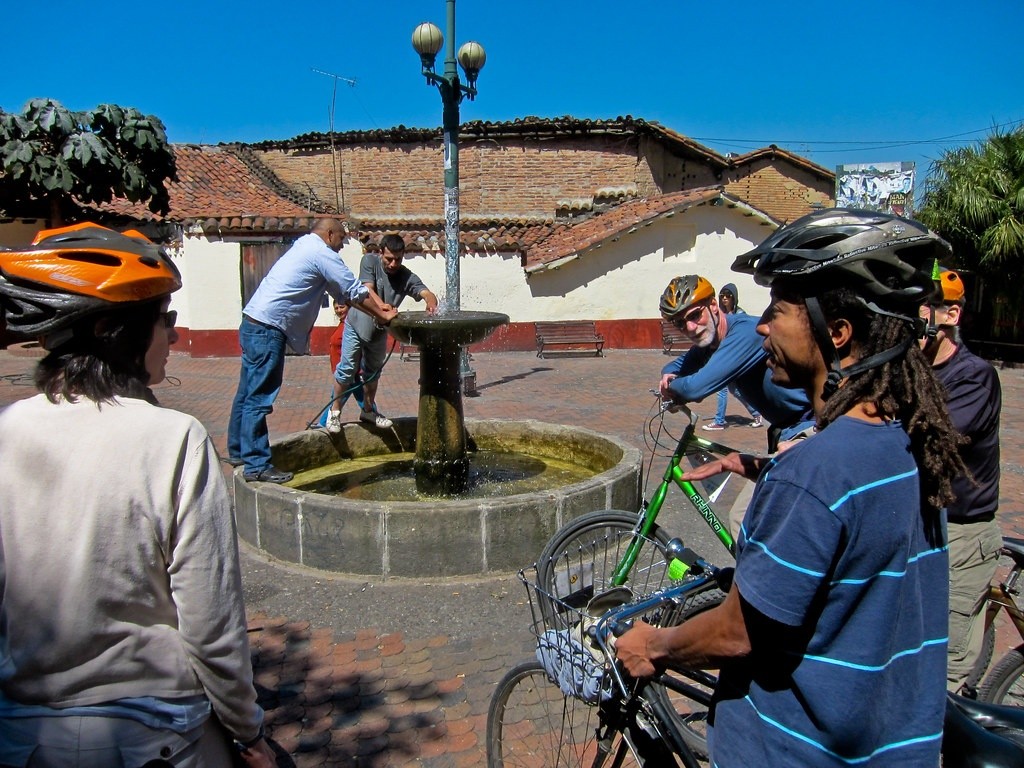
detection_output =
[243,466,294,484]
[229,457,244,466]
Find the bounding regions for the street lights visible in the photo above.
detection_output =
[411,21,486,310]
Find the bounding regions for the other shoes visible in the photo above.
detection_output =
[306,421,323,428]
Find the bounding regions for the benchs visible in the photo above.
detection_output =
[533,322,607,359]
[399,343,421,362]
[660,320,693,357]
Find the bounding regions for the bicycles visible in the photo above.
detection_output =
[535,389,770,629]
[673,519,1024,710]
[486,537,1024,768]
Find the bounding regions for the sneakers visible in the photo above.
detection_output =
[748,414,763,428]
[702,422,724,430]
[325,406,341,434]
[359,408,393,429]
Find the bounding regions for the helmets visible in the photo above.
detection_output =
[659,275,715,321]
[730,209,955,315]
[937,267,968,308]
[0,221,182,350]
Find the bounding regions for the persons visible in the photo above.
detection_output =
[228,215,399,481]
[701,282,763,432]
[658,274,819,454]
[907,267,999,701]
[616,209,950,768]
[0,206,281,768]
[306,234,439,435]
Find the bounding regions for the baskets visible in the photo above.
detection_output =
[516,531,709,708]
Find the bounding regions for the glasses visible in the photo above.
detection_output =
[719,294,732,298]
[157,310,177,329]
[671,304,708,328]
